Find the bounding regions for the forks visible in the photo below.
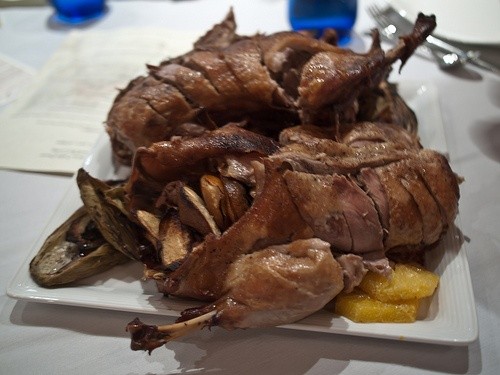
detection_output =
[366,3,458,70]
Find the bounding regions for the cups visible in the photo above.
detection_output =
[48,0,108,24]
[288,1,357,47]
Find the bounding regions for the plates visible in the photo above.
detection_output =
[6,73,479,345]
